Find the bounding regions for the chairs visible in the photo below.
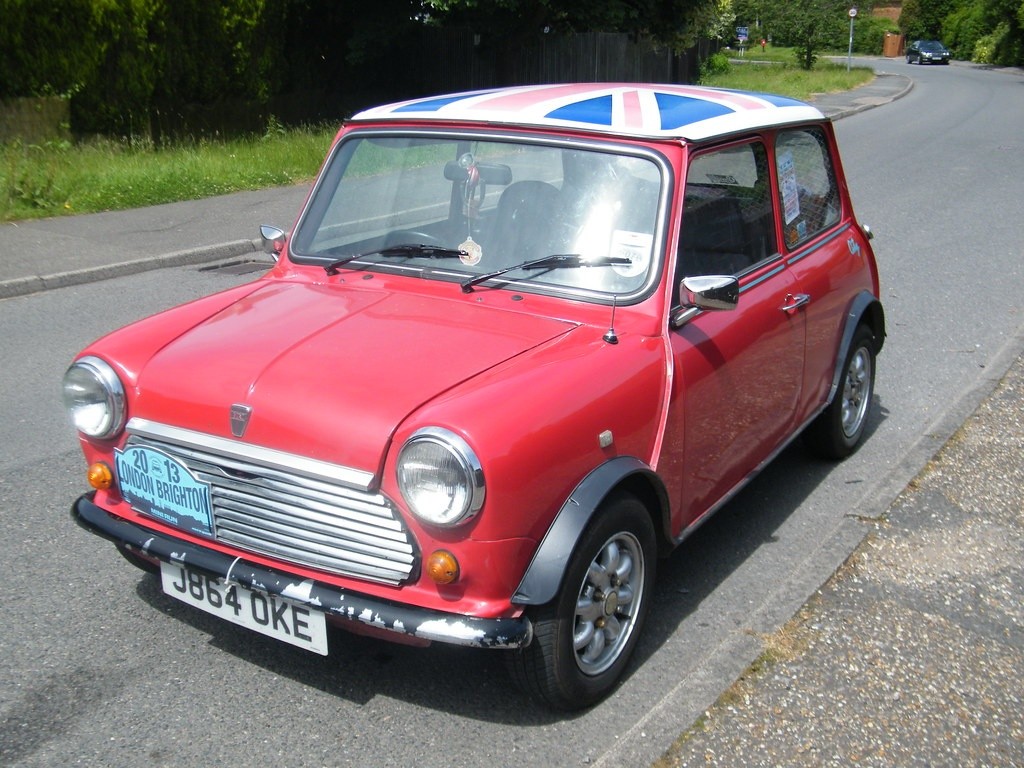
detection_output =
[676,197,749,285]
[491,180,572,262]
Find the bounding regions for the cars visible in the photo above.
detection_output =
[905,39,950,66]
[62,79,888,718]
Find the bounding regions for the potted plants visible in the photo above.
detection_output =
[884,28,904,56]
